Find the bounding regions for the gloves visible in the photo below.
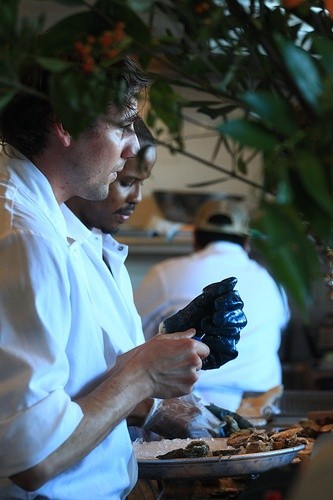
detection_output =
[158,277,248,370]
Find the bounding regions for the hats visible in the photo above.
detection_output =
[195,199,251,236]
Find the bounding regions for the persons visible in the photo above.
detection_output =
[0,41,247,500]
[133,198,291,414]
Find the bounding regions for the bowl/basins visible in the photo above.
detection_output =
[153,190,246,223]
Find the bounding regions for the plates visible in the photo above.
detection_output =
[137,445,305,477]
